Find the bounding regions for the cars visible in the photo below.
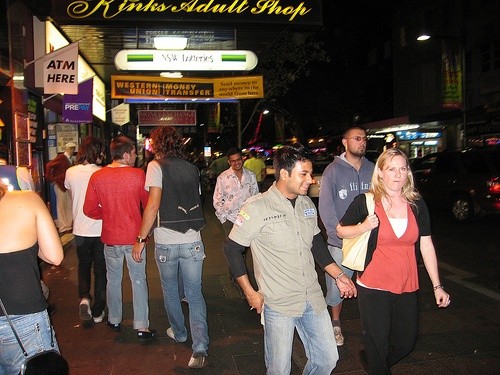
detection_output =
[411,151,500,224]
[308,160,332,197]
[264,157,274,175]
[409,157,422,164]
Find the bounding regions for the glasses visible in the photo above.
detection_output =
[229,155,243,163]
[346,136,368,141]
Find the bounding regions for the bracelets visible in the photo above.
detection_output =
[434,284,444,291]
[335,271,345,283]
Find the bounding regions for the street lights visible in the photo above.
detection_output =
[417,30,468,148]
[263,109,289,138]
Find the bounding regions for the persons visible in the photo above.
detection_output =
[336,149,450,374]
[64,139,105,323]
[226,143,357,375]
[319,127,375,346]
[83,136,158,341]
[0,119,35,191]
[213,148,258,283]
[243,149,266,191]
[210,151,231,188]
[131,125,209,368]
[0,180,64,375]
[45,143,75,235]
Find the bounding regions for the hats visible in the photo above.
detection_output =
[66,142,77,148]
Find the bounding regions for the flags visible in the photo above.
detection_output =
[63,77,93,123]
[44,42,78,94]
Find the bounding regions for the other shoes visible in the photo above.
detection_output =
[93,310,105,323]
[333,326,345,346]
[188,346,208,368]
[79,298,93,321]
[166,326,187,342]
[137,330,156,340]
[107,321,120,329]
[359,350,368,373]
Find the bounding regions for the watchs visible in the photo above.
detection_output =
[137,235,147,244]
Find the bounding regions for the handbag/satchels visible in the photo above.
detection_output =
[341,193,375,271]
[20,350,69,375]
